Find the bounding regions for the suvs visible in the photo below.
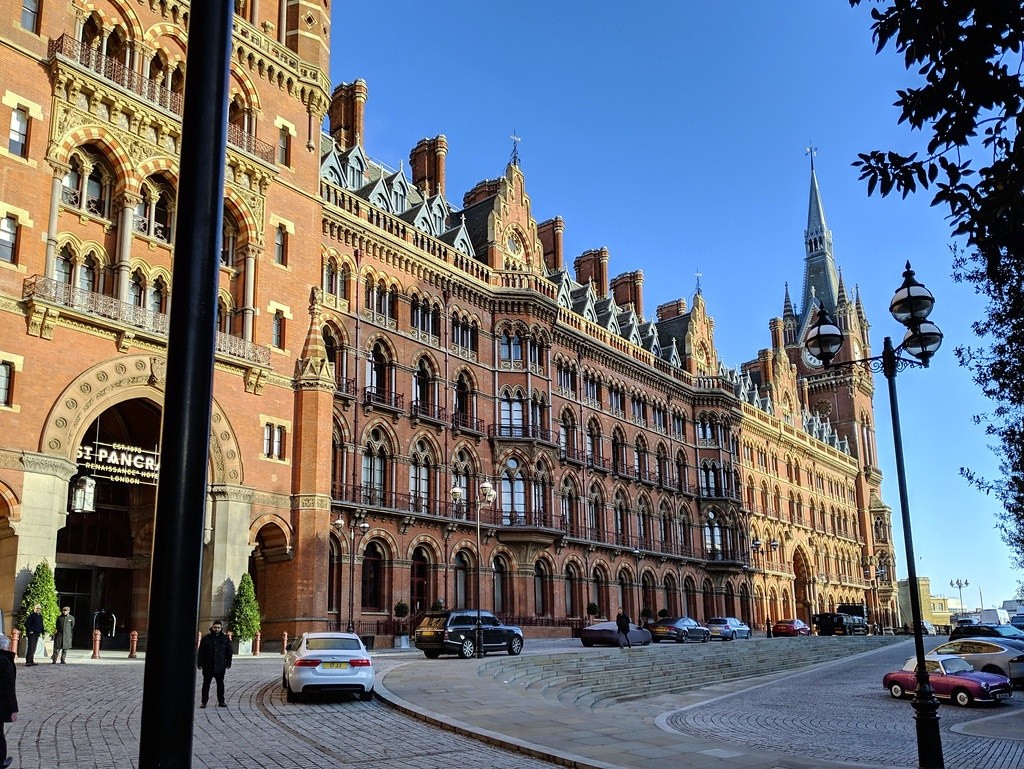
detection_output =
[414,609,525,659]
[1008,613,1024,632]
[948,618,1024,642]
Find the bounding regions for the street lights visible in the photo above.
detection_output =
[334,512,371,633]
[450,473,498,659]
[800,261,945,769]
[632,546,643,626]
[742,562,751,629]
[864,566,886,635]
[950,579,970,618]
[750,534,781,637]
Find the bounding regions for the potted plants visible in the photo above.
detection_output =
[225,572,261,657]
[393,600,409,649]
[18,558,61,662]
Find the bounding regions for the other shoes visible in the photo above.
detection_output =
[61,660,66,663]
[26,662,38,666]
[0,757,13,769]
[219,702,227,707]
[53,660,56,664]
[201,703,206,707]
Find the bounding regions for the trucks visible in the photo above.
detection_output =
[812,601,872,636]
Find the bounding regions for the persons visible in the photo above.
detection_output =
[197,620,233,708]
[748,619,752,637]
[52,607,74,664]
[879,621,883,635]
[873,621,877,636]
[904,622,909,635]
[616,607,631,649]
[812,624,818,637]
[24,604,45,666]
[0,632,19,769]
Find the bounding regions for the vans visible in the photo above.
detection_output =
[979,609,1011,625]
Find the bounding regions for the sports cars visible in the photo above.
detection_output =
[882,655,1014,708]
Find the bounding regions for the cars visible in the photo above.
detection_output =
[925,637,1024,686]
[772,619,811,638]
[910,621,937,636]
[706,618,751,640]
[580,621,652,647]
[282,633,375,703]
[648,617,710,643]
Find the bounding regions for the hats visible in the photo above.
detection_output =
[62,606,70,611]
